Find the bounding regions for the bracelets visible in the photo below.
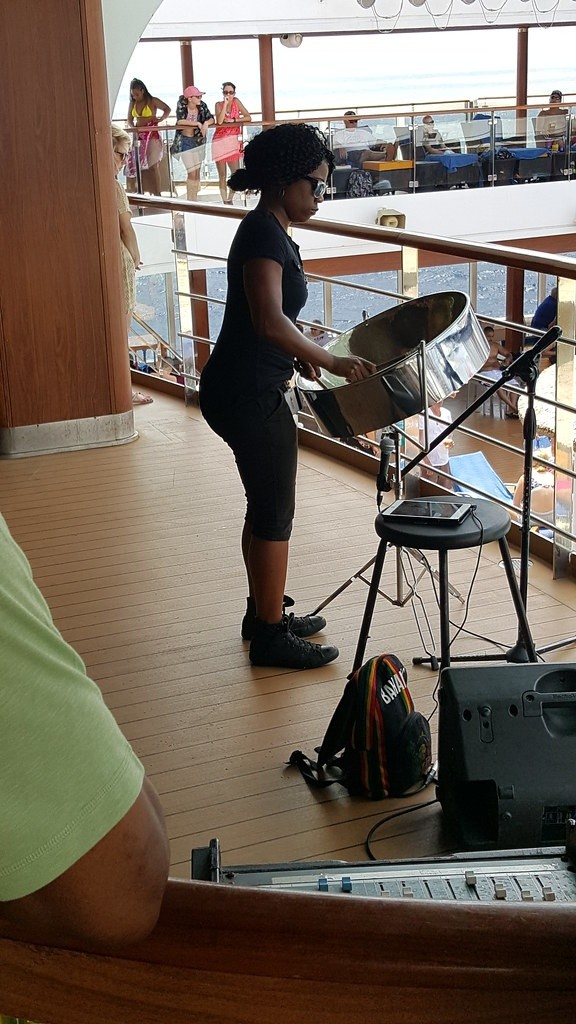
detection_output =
[233,117,236,122]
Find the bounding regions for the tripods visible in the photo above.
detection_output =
[394,325,576,666]
[308,423,466,618]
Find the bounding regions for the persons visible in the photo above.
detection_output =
[170,85,215,201]
[123,77,172,197]
[423,115,457,155]
[195,122,377,670]
[0,520,171,960]
[304,319,331,349]
[332,111,399,166]
[112,124,153,406]
[536,89,576,151]
[478,287,558,419]
[418,399,455,490]
[210,82,252,207]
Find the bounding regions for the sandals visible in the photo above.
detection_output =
[132,392,153,404]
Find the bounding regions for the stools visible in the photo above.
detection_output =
[346,496,537,680]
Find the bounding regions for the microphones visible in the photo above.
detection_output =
[376,439,395,505]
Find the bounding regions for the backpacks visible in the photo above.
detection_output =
[282,654,431,801]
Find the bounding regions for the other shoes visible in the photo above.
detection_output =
[386,143,395,161]
[393,141,398,160]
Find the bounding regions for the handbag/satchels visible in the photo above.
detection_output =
[532,435,551,449]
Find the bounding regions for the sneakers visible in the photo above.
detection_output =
[249,612,339,669]
[241,595,326,638]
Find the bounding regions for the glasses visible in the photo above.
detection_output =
[222,91,235,95]
[550,96,560,99]
[349,120,357,123]
[303,174,329,198]
[115,151,129,161]
[428,120,434,123]
[192,96,202,99]
[310,328,317,330]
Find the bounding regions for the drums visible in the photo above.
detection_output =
[293,287,492,440]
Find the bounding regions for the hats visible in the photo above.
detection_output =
[551,90,562,98]
[344,111,355,115]
[183,86,206,98]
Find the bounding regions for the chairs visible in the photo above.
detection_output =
[466,379,509,419]
[325,122,576,196]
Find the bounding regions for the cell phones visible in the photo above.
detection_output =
[382,500,471,523]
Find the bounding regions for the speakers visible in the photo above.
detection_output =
[434,661,576,851]
